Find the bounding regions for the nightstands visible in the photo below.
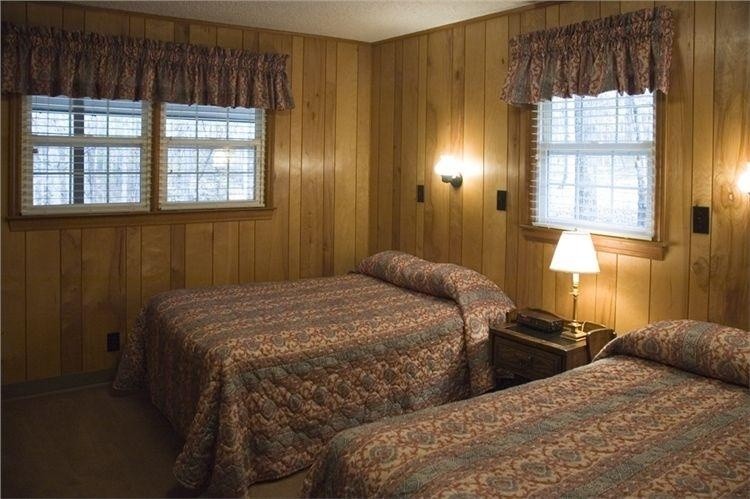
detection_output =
[488,322,591,390]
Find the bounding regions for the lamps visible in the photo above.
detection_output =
[549,231,601,342]
[433,156,463,187]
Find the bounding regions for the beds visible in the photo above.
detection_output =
[297,355,750,499]
[110,273,469,498]
